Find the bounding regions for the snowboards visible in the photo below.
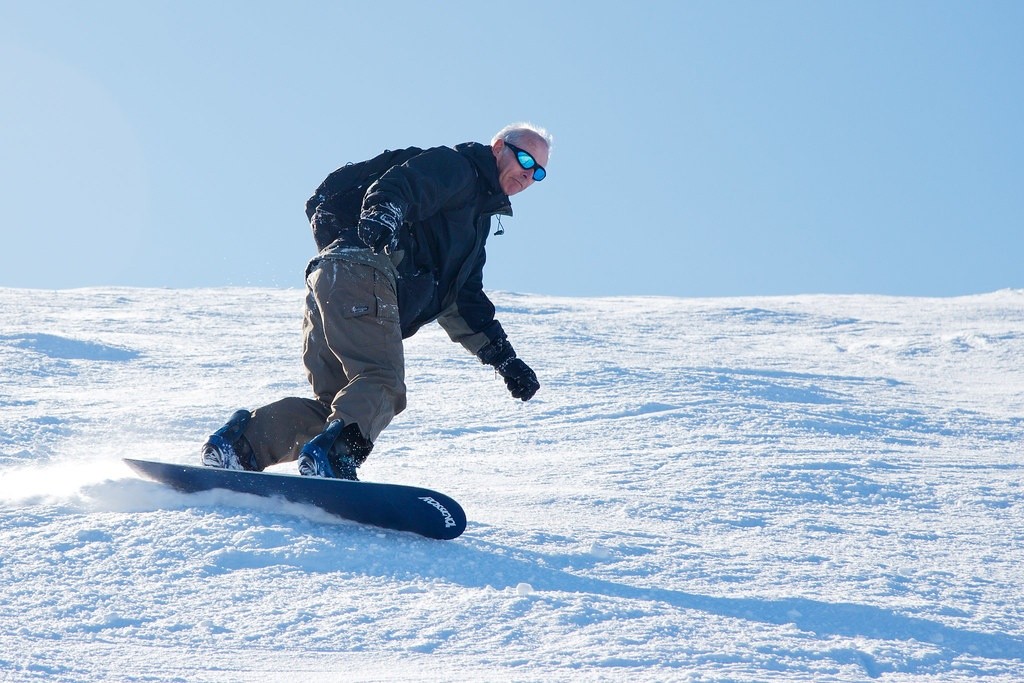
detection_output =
[121,458,468,540]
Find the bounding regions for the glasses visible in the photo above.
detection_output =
[504,141,546,181]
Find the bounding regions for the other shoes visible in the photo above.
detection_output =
[203,409,262,471]
[299,421,365,481]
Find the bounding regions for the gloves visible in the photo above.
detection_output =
[504,358,540,401]
[358,198,404,255]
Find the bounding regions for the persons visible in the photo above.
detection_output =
[202,124,554,480]
